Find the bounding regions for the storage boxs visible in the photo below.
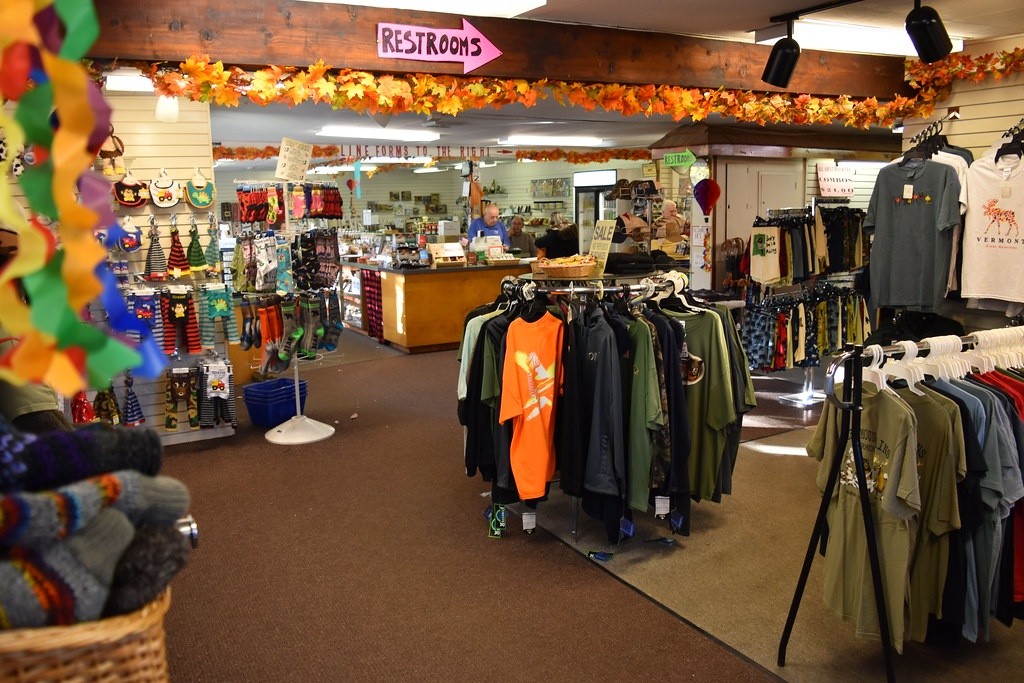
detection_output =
[242,378,308,427]
[0,583,174,682]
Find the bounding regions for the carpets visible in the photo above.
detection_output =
[503,422,1024,682]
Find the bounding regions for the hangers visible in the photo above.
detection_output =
[815,326,1024,419]
[744,280,862,320]
[466,271,730,342]
[877,120,973,192]
[969,119,1023,178]
[747,198,867,238]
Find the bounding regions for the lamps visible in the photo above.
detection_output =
[904,0,953,63]
[761,17,806,91]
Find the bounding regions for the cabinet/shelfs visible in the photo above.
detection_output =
[572,168,642,258]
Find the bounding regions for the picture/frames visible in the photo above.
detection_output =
[367,188,453,221]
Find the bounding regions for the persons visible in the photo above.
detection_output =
[468,204,510,252]
[506,216,537,258]
[534,212,579,259]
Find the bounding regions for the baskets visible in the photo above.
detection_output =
[243,377,309,428]
[2,584,170,681]
